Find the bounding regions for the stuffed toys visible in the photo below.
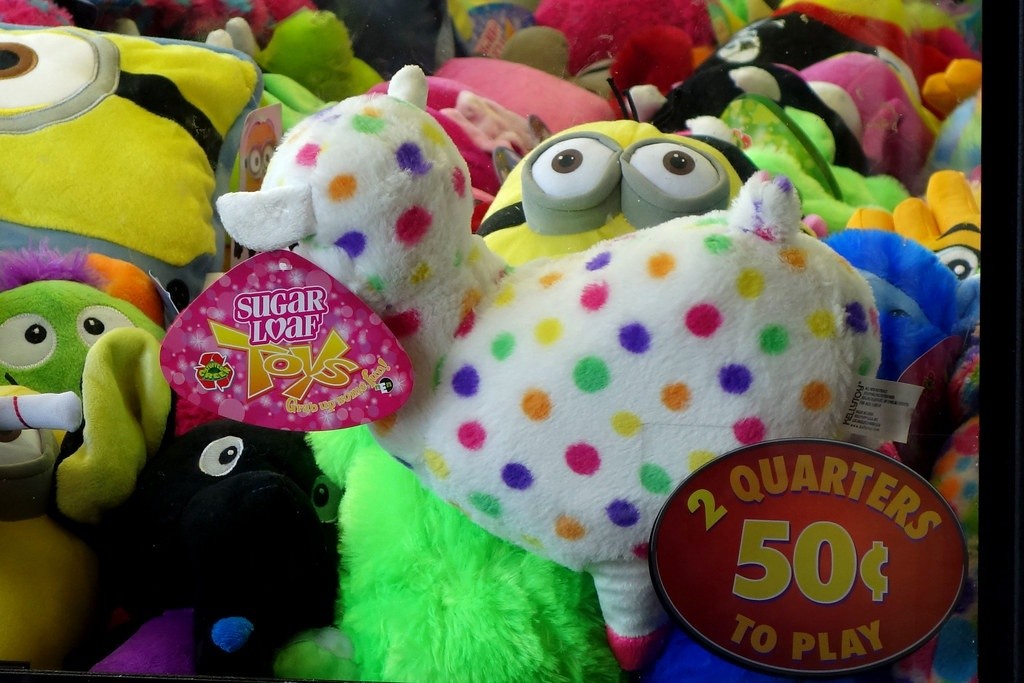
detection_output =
[0,0,984,683]
[214,65,903,667]
